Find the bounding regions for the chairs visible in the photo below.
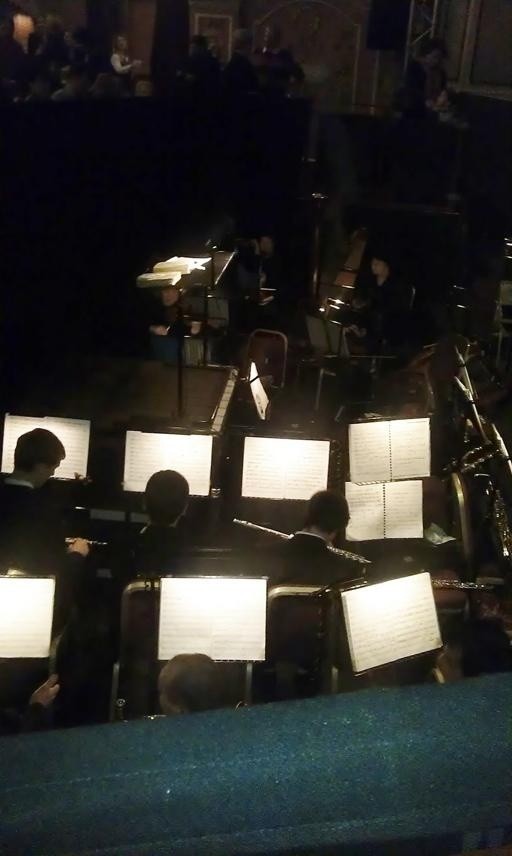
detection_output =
[422,471,476,574]
[405,284,415,311]
[294,296,345,413]
[494,279,512,368]
[109,581,253,720]
[266,585,334,702]
[239,328,288,392]
[426,588,471,684]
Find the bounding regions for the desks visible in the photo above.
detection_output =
[85,358,240,505]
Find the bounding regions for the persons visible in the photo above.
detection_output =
[401,34,449,120]
[114,470,192,577]
[1,9,307,99]
[1,428,98,575]
[265,490,371,589]
[343,251,409,377]
[157,653,227,717]
[0,672,62,733]
